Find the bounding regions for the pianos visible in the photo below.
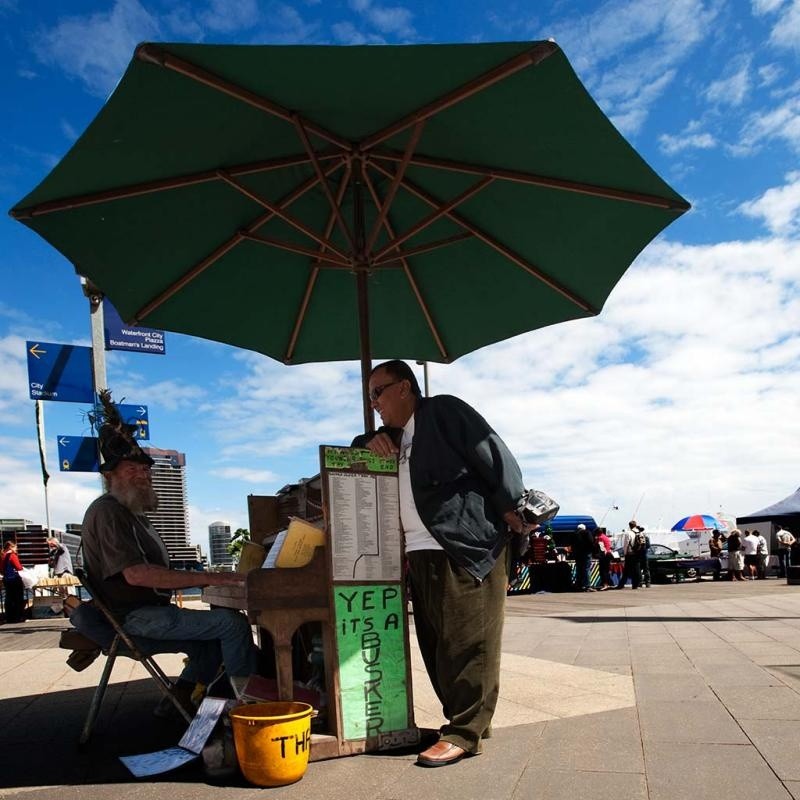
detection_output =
[201,444,421,762]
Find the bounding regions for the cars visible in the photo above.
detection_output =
[613,543,701,585]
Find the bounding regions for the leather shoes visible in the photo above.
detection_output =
[418,740,466,767]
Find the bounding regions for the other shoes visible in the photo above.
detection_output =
[713,573,768,581]
[632,586,637,588]
[615,584,624,589]
[601,586,608,590]
[154,682,198,732]
[638,584,642,587]
[587,588,597,592]
[646,583,650,587]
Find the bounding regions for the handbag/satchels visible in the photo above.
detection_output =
[4,553,17,578]
[516,489,558,525]
[646,537,650,550]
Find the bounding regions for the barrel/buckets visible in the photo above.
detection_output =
[228,701,318,789]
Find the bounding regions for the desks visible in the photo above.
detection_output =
[31,584,84,619]
[656,558,721,583]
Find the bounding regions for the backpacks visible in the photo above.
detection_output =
[630,530,640,551]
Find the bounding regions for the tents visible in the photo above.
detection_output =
[736,486,800,577]
[536,515,599,561]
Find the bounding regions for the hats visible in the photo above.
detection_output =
[77,388,155,473]
[577,524,585,530]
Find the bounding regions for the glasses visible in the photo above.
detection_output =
[373,382,394,400]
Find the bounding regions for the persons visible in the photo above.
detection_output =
[636,527,651,588]
[752,529,768,579]
[573,524,597,592]
[728,529,749,581]
[349,360,541,768]
[0,540,24,623]
[593,527,614,591]
[742,528,760,579]
[81,424,258,730]
[709,530,723,580]
[613,521,643,589]
[775,524,796,577]
[46,537,74,615]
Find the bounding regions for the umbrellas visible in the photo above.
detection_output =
[671,514,726,558]
[7,39,694,434]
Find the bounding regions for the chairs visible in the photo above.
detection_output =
[73,568,195,746]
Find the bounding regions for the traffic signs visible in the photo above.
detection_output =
[26,340,95,402]
[57,435,102,472]
[94,403,150,440]
